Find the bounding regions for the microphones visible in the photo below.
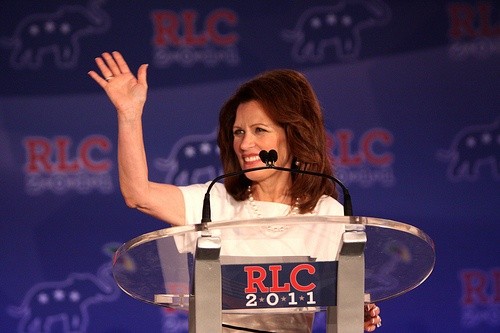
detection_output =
[200,149,353,223]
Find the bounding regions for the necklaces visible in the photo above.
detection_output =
[247,183,300,233]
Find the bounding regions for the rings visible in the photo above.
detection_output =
[105,76,113,80]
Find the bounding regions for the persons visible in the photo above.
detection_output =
[86,50,384,333]
